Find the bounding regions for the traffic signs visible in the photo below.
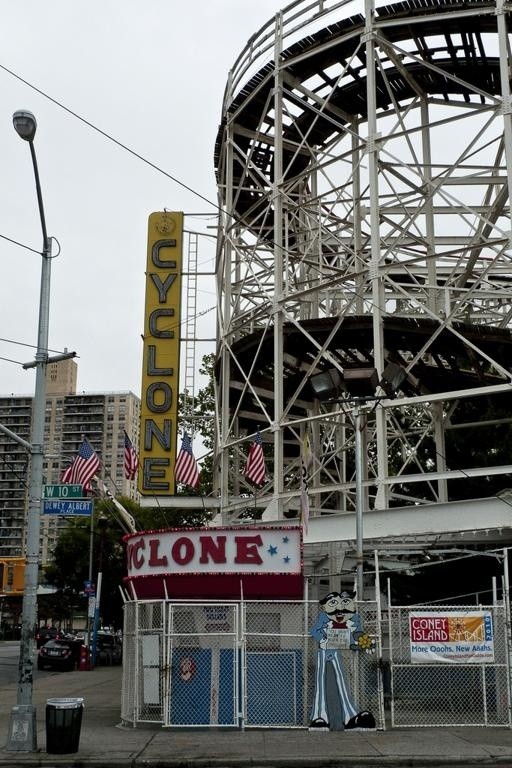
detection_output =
[41,500,93,516]
[42,484,83,499]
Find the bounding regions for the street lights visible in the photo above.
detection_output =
[9,110,55,752]
[307,360,410,711]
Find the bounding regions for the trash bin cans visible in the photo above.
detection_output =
[46,698,85,755]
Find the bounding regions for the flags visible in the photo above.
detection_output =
[59,435,102,495]
[122,433,140,481]
[300,432,311,535]
[173,431,199,489]
[92,475,137,533]
[245,430,265,487]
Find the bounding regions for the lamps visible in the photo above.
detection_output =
[308,362,409,432]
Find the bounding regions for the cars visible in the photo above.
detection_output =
[37,626,122,672]
